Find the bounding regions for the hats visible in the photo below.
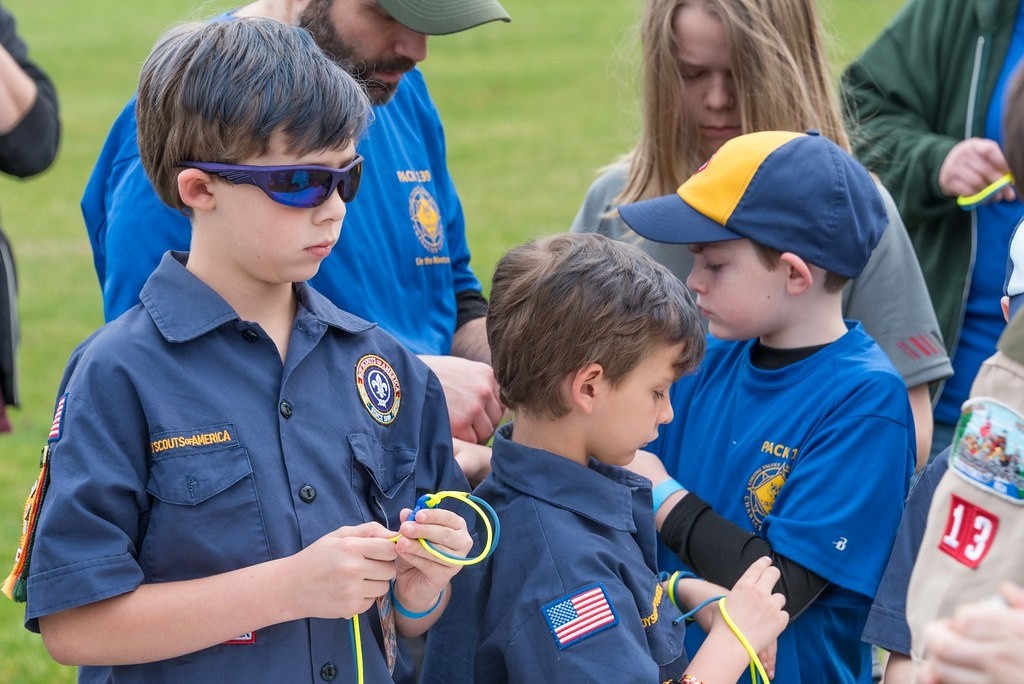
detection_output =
[616,129,890,277]
[1007,220,1024,297]
[377,0,511,35]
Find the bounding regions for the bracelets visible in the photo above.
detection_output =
[956,174,1012,211]
[419,491,500,566]
[390,580,444,618]
[668,570,697,621]
[653,478,685,514]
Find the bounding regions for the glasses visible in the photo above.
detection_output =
[174,153,364,207]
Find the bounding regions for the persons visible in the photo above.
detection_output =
[452,131,917,684]
[568,0,953,473]
[418,232,789,684]
[836,0,1024,466]
[81,0,512,447]
[0,17,476,684]
[0,8,62,431]
[859,57,1024,684]
